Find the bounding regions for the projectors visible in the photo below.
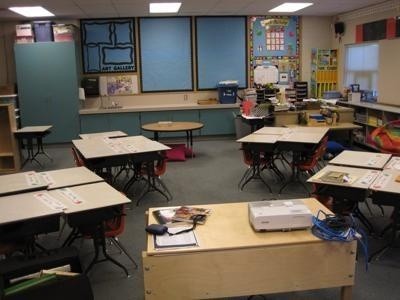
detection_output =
[248,200,313,232]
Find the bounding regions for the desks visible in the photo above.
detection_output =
[141,197,357,300]
[0,165,132,277]
[13,126,52,169]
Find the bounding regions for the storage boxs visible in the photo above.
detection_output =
[217,83,238,103]
[16,21,79,42]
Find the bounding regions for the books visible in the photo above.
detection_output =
[9,264,71,285]
[152,226,199,252]
[173,205,210,225]
[152,208,180,224]
[4,272,56,293]
[2,275,58,298]
[322,170,348,183]
[39,269,81,282]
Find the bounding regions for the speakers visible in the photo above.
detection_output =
[80,76,99,98]
[334,22,344,33]
